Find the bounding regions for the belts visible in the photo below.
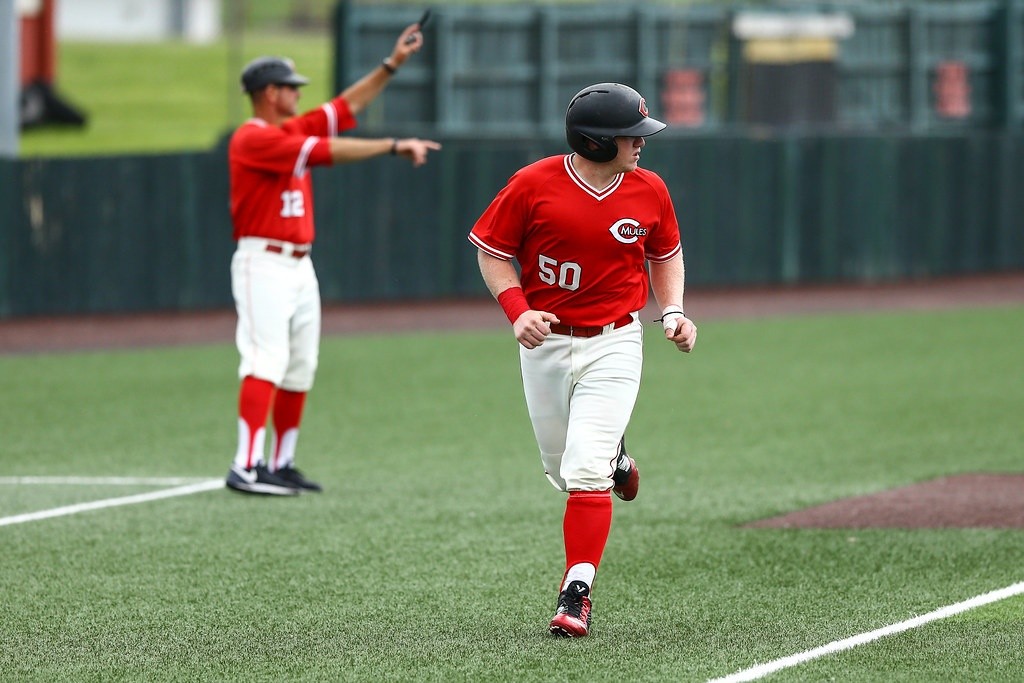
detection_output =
[547,314,632,337]
[266,245,310,258]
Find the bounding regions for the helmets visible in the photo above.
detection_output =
[242,56,309,87]
[566,83,668,163]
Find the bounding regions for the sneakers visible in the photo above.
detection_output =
[226,459,299,495]
[613,434,639,501]
[272,460,322,493]
[548,580,593,636]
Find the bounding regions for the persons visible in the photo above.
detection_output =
[225,23,442,497]
[469,82,697,640]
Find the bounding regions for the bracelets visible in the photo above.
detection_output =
[662,304,684,324]
[653,311,686,323]
[391,140,398,156]
[382,58,398,75]
